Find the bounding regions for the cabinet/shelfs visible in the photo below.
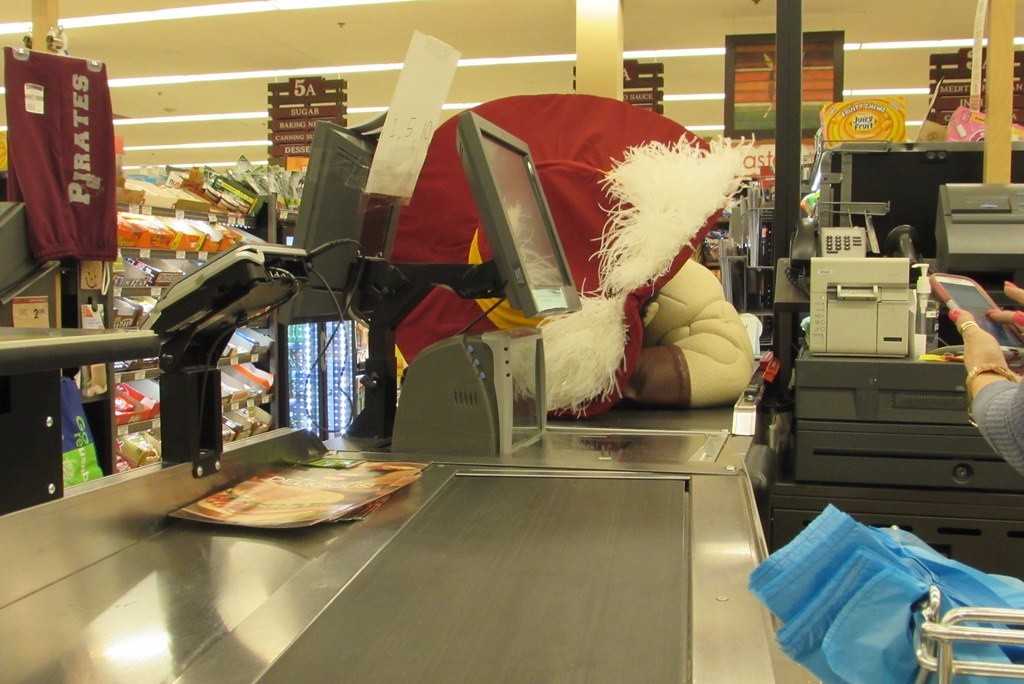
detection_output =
[103,192,281,475]
[738,206,775,357]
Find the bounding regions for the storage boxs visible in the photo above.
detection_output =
[221,362,275,404]
[113,296,158,328]
[112,259,206,287]
[116,157,265,217]
[113,379,160,426]
[221,405,273,444]
[115,427,162,472]
[223,328,276,358]
[114,212,241,253]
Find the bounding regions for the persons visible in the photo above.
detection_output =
[387,93,755,417]
[948,281,1024,474]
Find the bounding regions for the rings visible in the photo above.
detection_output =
[959,321,978,333]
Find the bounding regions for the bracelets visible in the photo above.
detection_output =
[966,363,1018,402]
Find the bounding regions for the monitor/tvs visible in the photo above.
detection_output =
[456,110,583,319]
[277,120,400,325]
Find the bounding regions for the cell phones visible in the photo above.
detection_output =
[930,272,1024,360]
